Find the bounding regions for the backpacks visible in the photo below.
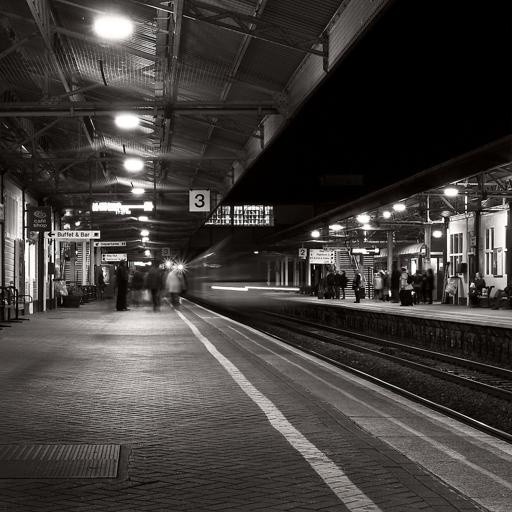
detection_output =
[406,272,415,284]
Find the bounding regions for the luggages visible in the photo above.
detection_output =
[317,283,323,299]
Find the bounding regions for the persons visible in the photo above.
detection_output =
[98,257,188,314]
[468,271,486,309]
[294,260,435,307]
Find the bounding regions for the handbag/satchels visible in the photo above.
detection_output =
[324,292,331,299]
